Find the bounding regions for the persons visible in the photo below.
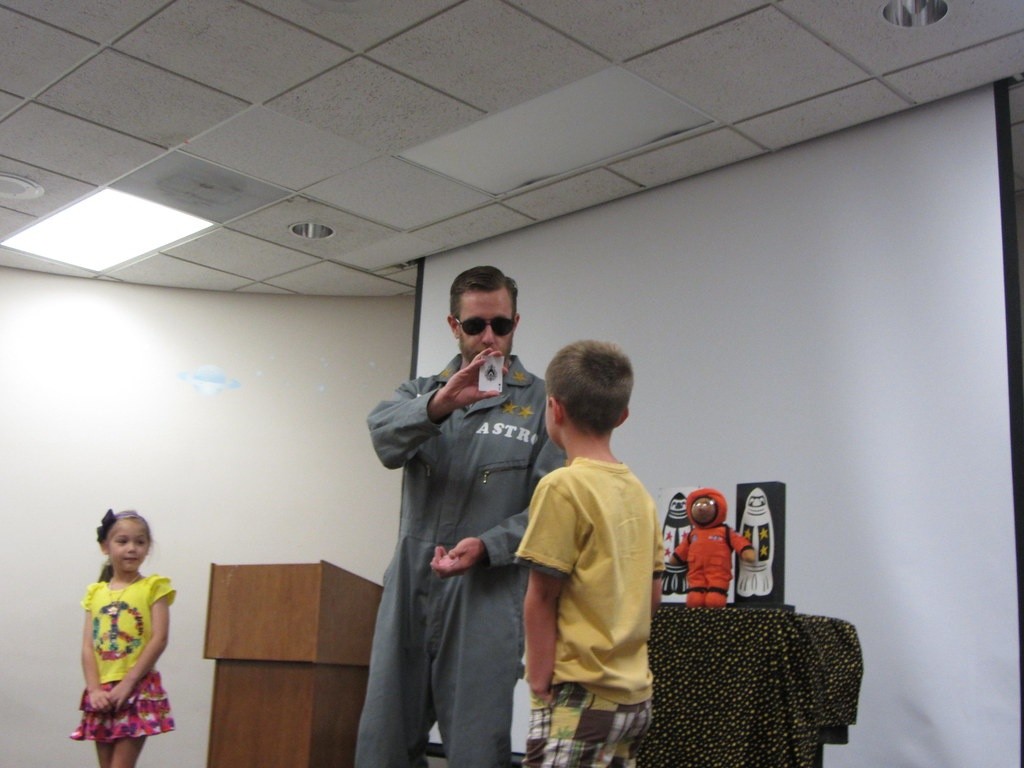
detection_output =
[71,509,176,768]
[514,340,666,768]
[355,265,567,768]
[670,488,756,607]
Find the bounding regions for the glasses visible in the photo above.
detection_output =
[455,316,515,336]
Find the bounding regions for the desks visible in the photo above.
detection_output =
[639,606,864,768]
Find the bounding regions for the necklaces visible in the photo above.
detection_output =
[108,575,140,616]
